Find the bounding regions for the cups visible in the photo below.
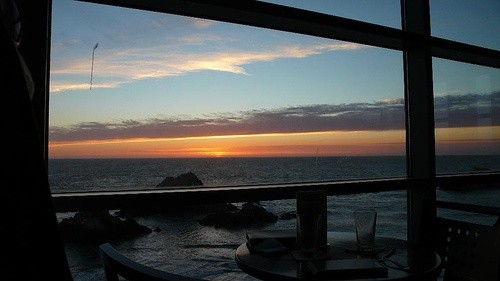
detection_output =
[353,212,377,251]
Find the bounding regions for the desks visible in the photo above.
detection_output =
[235,229,442,281]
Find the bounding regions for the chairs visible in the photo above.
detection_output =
[415,196,500,281]
